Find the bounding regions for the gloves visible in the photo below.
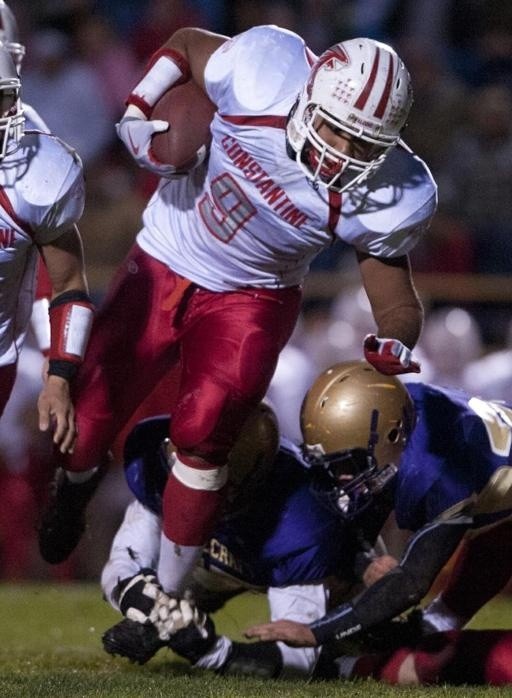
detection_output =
[114,117,190,181]
[362,333,423,375]
[156,597,219,665]
[110,567,168,622]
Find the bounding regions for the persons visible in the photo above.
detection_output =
[244,359,512,685]
[1,0,512,580]
[42,24,438,660]
[100,402,390,677]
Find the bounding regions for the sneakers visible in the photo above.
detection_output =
[355,607,432,653]
[37,449,115,566]
[101,617,162,665]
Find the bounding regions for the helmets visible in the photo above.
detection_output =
[284,37,415,194]
[164,399,280,519]
[1,2,28,163]
[302,360,417,529]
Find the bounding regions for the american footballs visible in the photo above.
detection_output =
[150,83,216,175]
